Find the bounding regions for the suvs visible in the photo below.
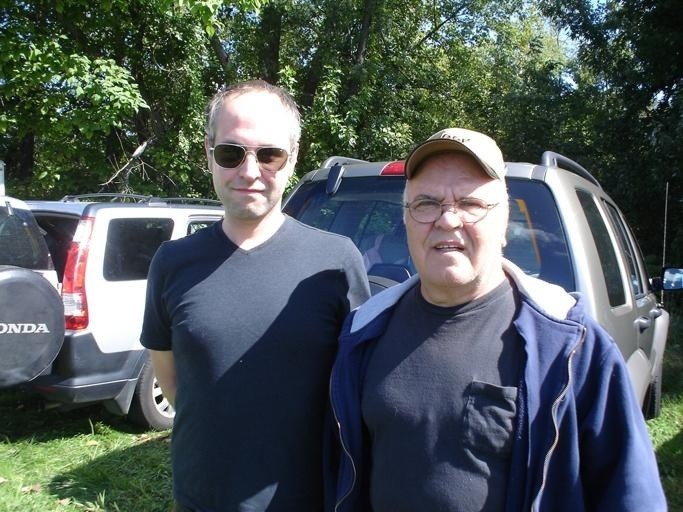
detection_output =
[28,192,225,431]
[281,143,682,424]
[0,194,66,389]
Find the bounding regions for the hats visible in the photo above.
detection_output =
[405,127,504,180]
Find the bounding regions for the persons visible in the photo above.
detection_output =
[329,128,666,512]
[141,80,369,512]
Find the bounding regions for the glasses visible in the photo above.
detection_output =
[401,197,499,223]
[209,143,292,172]
